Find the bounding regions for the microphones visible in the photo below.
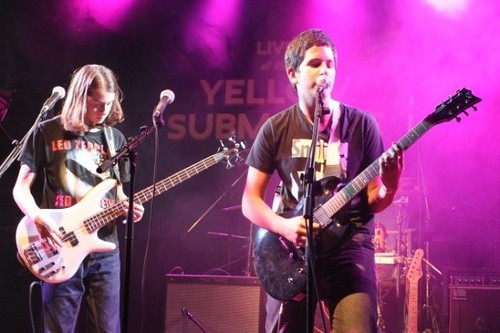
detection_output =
[152,89,175,117]
[41,86,65,110]
[316,76,332,93]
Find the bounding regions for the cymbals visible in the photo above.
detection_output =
[400,178,419,188]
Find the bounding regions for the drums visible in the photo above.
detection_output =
[373,222,388,253]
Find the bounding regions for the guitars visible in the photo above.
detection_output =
[253,87,482,301]
[405,248,423,333]
[16,136,249,284]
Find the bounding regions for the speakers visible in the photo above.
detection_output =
[439,288,500,333]
[156,273,267,333]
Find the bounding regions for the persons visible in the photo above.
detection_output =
[13,65,144,333]
[242,28,404,333]
